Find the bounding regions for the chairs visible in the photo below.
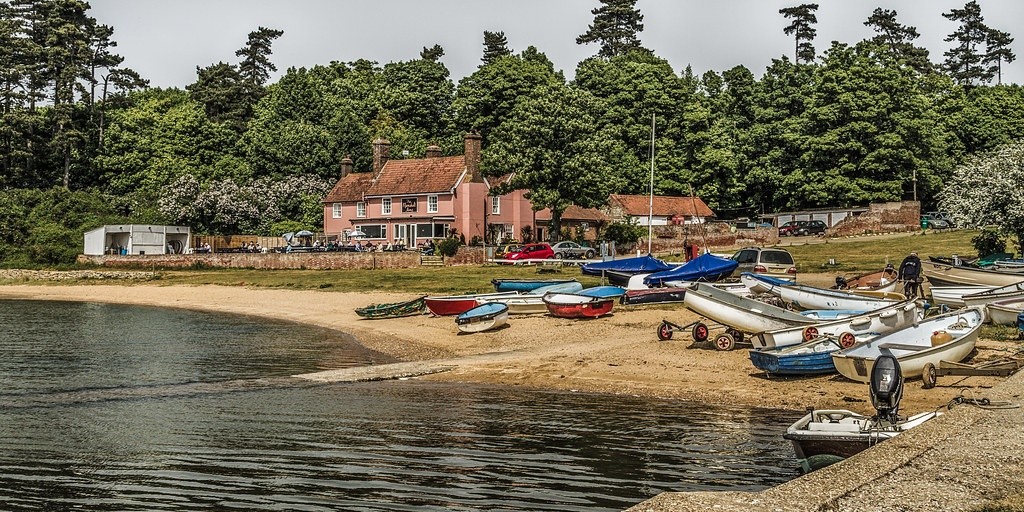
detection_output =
[808,423,860,433]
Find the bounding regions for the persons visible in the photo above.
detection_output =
[347,240,352,246]
[393,239,404,245]
[355,242,362,252]
[241,241,262,253]
[201,242,212,254]
[306,238,312,252]
[365,241,373,252]
[313,239,343,252]
[375,241,383,252]
[898,252,921,298]
[385,241,393,251]
[422,239,435,256]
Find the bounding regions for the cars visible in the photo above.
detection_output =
[920,210,968,229]
[794,220,828,235]
[729,247,797,282]
[495,241,597,267]
[778,220,805,236]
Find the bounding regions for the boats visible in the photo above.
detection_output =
[454,302,509,333]
[424,277,700,316]
[354,295,429,318]
[784,405,942,469]
[542,290,614,317]
[684,254,1024,375]
[831,306,984,385]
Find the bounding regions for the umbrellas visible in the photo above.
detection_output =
[348,231,366,237]
[296,230,313,244]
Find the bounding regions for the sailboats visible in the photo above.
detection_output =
[581,114,738,287]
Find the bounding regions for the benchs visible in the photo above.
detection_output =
[750,308,764,313]
[857,287,869,289]
[512,301,520,303]
[695,289,712,296]
[879,343,931,350]
[941,329,971,338]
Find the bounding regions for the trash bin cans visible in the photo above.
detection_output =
[920,217,928,229]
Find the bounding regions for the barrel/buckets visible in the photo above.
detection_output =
[931,330,956,347]
